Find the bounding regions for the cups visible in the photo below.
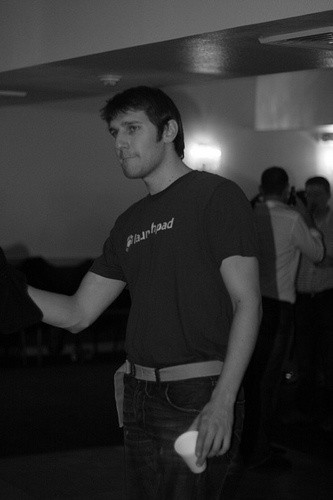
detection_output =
[173,429,207,475]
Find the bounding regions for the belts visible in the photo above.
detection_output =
[114,360,225,428]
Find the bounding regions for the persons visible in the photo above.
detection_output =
[24,84,264,500]
[239,166,326,463]
[293,176,333,459]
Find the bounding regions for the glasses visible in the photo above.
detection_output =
[303,191,326,198]
